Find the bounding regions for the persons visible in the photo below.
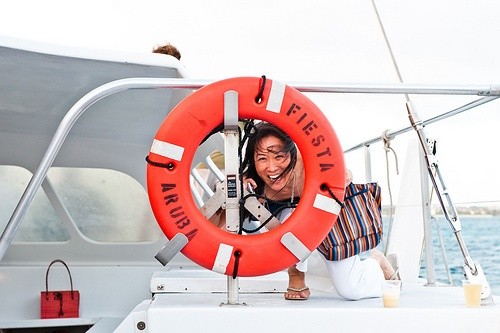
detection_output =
[207,122,404,300]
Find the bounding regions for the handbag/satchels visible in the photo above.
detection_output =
[317,183,383,261]
[41,259,80,319]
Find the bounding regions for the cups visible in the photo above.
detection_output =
[461,280,482,309]
[382,280,401,307]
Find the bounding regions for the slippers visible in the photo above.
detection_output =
[385,253,402,294]
[284,286,310,299]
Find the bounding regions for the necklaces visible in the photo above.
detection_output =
[291,170,301,203]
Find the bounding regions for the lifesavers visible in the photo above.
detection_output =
[146,77,345,277]
